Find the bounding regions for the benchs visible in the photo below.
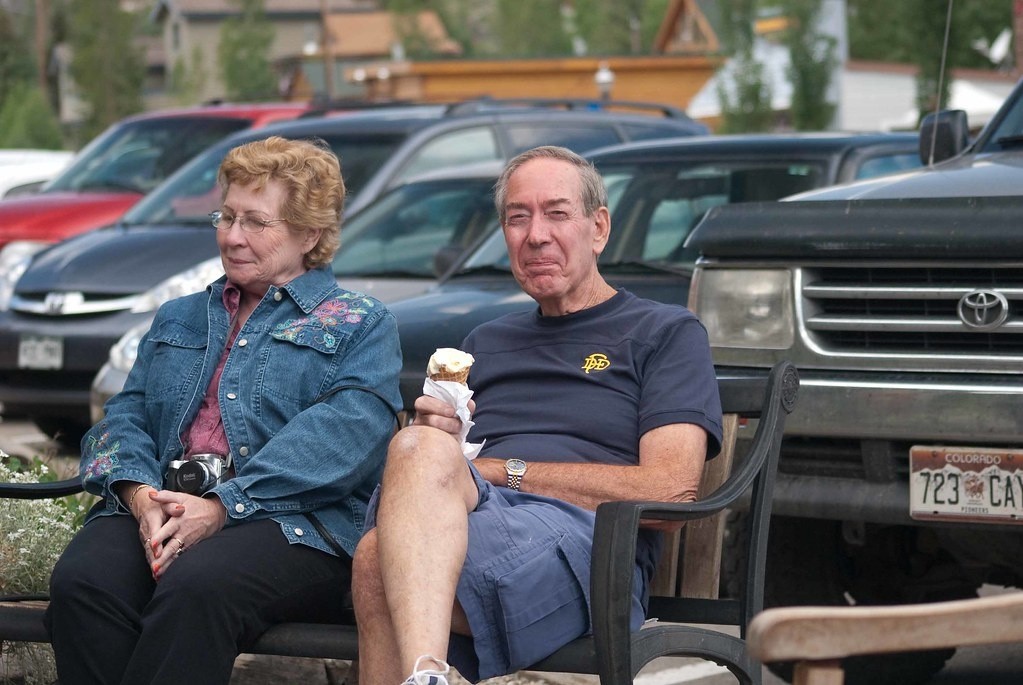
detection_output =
[0,361,801,685]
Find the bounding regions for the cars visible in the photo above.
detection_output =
[329,158,512,309]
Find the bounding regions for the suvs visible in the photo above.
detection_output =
[0,93,716,453]
[376,130,931,426]
[682,78,1023,685]
[0,93,349,318]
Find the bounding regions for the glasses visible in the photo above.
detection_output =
[208,210,287,234]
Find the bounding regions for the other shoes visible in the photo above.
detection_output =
[399,655,450,685]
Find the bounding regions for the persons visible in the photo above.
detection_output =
[44,134,407,685]
[350,145,725,685]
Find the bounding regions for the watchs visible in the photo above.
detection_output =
[503,458,527,490]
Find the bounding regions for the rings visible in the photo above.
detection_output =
[144,538,150,544]
[176,549,183,556]
[175,538,185,548]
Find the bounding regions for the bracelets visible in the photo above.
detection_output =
[129,485,148,510]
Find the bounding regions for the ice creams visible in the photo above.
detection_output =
[426,348,475,386]
